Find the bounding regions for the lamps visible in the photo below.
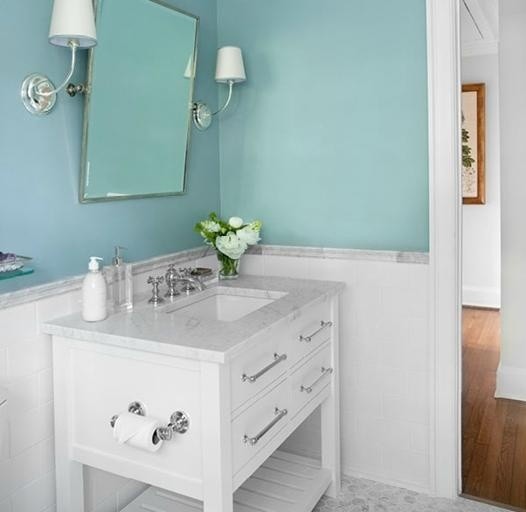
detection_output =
[19,0,97,117]
[192,46,247,132]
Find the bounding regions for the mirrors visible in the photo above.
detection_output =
[78,0,201,204]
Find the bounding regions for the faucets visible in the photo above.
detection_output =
[164,264,207,298]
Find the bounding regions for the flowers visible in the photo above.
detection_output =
[193,211,264,275]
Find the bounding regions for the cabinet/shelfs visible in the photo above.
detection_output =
[51,291,341,511]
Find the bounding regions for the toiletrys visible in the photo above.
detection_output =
[82,256,108,322]
[103,245,135,315]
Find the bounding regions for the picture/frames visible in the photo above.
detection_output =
[461,82,487,205]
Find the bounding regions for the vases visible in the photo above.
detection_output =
[216,251,241,280]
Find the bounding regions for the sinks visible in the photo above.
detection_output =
[156,286,290,321]
